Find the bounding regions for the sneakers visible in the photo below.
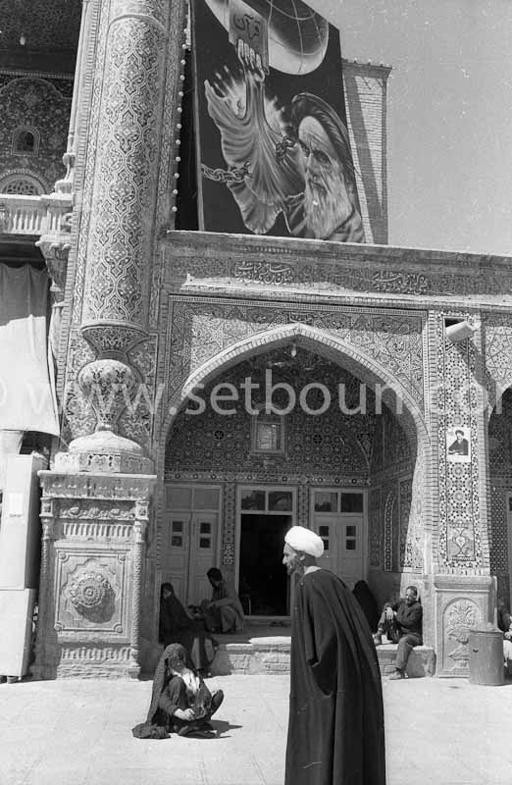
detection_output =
[374,635,382,645]
[390,670,408,680]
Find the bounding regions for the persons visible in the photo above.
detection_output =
[277,525,388,785]
[132,643,226,740]
[349,581,379,634]
[448,430,468,455]
[372,586,424,680]
[200,566,246,633]
[204,36,366,245]
[160,582,217,678]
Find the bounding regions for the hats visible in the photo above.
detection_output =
[285,527,325,558]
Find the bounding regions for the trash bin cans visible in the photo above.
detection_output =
[469,628,504,686]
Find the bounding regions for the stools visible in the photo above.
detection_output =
[242,592,257,615]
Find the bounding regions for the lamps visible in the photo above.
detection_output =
[290,337,297,357]
